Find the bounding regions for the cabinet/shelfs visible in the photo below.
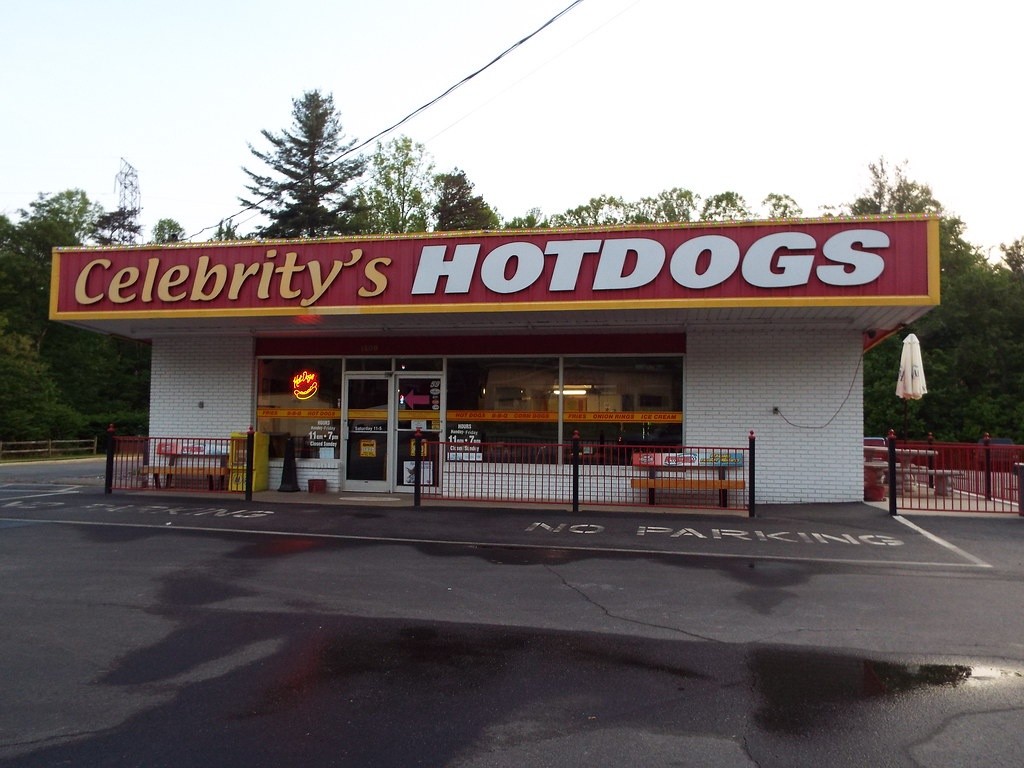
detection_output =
[227,431,269,492]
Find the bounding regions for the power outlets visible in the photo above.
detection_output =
[773,405,778,414]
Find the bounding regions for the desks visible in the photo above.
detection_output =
[863,446,938,491]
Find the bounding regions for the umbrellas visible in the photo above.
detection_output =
[896,334,927,447]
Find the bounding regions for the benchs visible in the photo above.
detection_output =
[881,469,966,498]
[138,444,231,491]
[866,460,927,485]
[631,452,747,508]
